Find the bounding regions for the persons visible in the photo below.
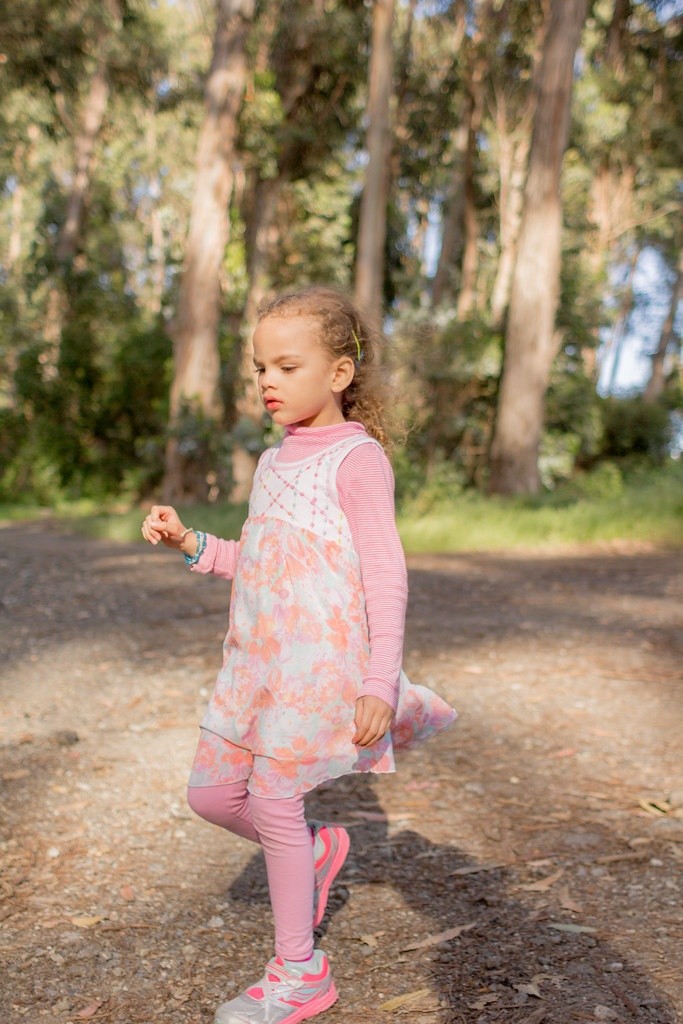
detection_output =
[141,286,460,1024]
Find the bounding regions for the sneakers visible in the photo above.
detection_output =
[307,818,350,929]
[215,948,338,1024]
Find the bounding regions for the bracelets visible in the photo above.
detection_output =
[175,527,194,549]
